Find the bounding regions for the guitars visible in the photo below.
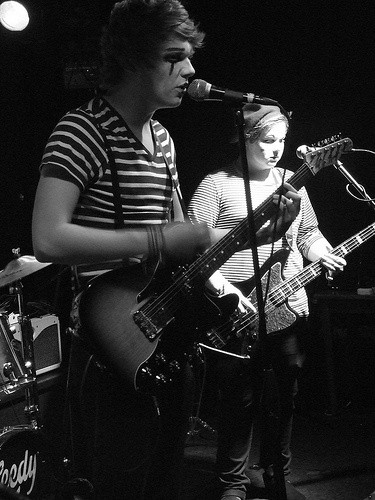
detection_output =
[65,131,354,390]
[198,221,375,360]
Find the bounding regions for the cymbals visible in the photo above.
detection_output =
[0,255,54,288]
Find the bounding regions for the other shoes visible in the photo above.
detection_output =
[223,487,248,500]
[267,482,305,500]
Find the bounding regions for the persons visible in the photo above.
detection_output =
[187,102,347,500]
[32,0,257,500]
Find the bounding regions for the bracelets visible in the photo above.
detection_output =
[146,225,166,256]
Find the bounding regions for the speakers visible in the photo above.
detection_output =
[0,372,62,500]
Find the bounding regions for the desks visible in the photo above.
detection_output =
[312,290,375,405]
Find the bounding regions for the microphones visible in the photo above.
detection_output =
[188,79,278,106]
[296,145,355,159]
